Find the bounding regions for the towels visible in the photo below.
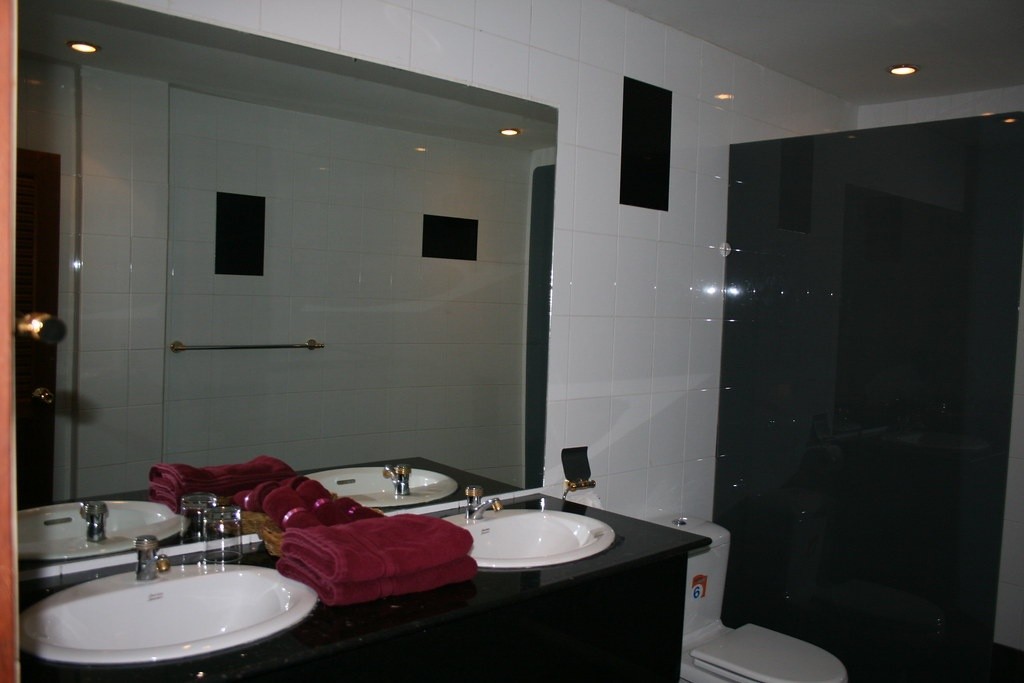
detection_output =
[148,483,271,515]
[277,556,479,604]
[146,456,294,497]
[282,514,475,583]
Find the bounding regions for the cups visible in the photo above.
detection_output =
[201,505,242,563]
[180,493,218,538]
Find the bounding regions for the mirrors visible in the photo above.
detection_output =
[15,0,558,576]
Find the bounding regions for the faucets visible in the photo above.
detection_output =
[79,501,107,541]
[134,534,171,581]
[382,462,411,496]
[464,484,504,519]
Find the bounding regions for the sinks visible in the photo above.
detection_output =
[17,500,190,559]
[304,466,459,507]
[439,509,616,568]
[20,563,318,663]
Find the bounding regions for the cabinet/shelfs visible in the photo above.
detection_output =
[240,555,689,682]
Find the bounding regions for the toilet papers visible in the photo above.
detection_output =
[565,479,603,509]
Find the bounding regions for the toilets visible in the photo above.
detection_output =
[647,517,849,683]
